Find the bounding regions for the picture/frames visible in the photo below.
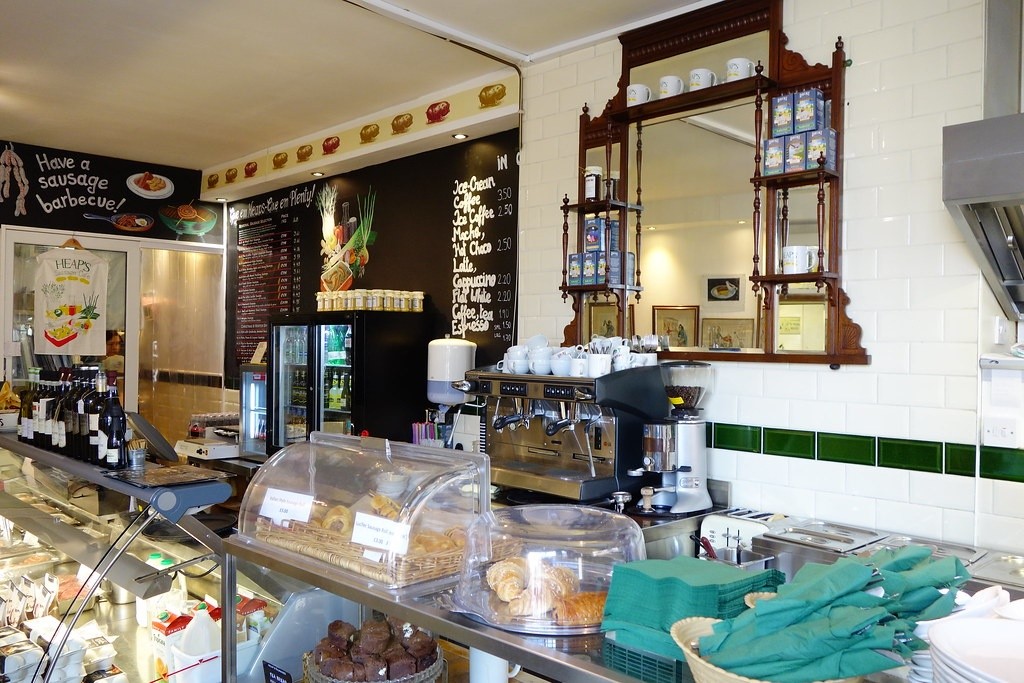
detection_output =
[700,274,746,314]
[588,302,635,343]
[652,305,699,346]
[701,318,755,348]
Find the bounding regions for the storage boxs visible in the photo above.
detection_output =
[627,251,636,286]
[763,88,837,178]
[452,413,481,453]
[599,637,696,683]
[171,629,263,683]
[569,217,623,285]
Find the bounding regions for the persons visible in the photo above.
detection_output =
[95,330,125,386]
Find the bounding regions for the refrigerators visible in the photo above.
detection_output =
[268,309,428,459]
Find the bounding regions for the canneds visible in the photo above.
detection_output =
[316,288,424,313]
[601,171,619,202]
[585,165,602,203]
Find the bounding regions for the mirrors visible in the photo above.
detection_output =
[559,0,874,370]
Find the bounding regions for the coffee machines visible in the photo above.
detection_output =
[639,361,713,514]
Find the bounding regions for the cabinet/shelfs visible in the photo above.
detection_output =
[628,120,645,303]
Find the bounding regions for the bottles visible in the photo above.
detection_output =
[603,171,619,201]
[284,324,352,447]
[17,368,126,469]
[135,553,187,627]
[585,166,602,203]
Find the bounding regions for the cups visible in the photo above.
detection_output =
[497,334,658,378]
[689,70,717,91]
[808,246,824,272]
[626,84,651,107]
[660,76,684,99]
[128,450,146,466]
[782,246,816,274]
[726,58,755,82]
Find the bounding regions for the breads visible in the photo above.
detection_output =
[311,490,469,554]
[486,557,609,625]
[314,613,439,682]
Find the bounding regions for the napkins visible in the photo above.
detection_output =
[597,544,972,683]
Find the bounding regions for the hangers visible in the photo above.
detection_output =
[60,230,86,251]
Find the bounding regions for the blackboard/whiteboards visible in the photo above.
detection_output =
[222,126,519,445]
